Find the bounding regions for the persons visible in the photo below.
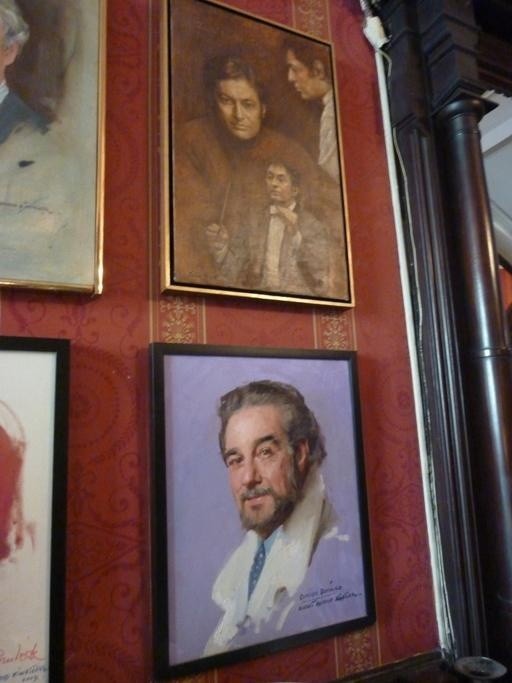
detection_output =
[200,378,339,662]
[204,159,332,300]
[282,35,339,184]
[0,1,38,145]
[173,42,347,299]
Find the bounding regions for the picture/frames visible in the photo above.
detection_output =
[146,340,376,682]
[0,0,109,301]
[2,333,73,682]
[151,0,357,309]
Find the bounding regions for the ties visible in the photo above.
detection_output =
[247,542,267,599]
